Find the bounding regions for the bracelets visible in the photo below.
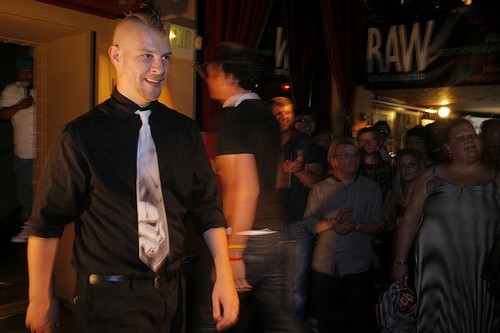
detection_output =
[295,166,303,173]
[227,244,247,249]
[393,259,408,265]
[229,256,244,261]
[354,222,360,232]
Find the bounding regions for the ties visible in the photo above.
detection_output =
[136,109,169,271]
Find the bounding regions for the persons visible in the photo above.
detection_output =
[373,148,429,296]
[292,103,500,202]
[393,119,500,333]
[0,54,38,243]
[25,8,239,333]
[301,137,388,333]
[269,95,324,333]
[205,49,299,333]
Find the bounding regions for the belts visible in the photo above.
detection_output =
[89,273,152,283]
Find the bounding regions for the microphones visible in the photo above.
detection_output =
[23,81,31,97]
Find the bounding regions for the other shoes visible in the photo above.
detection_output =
[12,219,31,242]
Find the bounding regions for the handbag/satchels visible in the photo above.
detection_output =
[376,276,417,332]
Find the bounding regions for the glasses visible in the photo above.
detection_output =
[335,152,360,157]
[380,129,390,133]
[295,117,315,122]
